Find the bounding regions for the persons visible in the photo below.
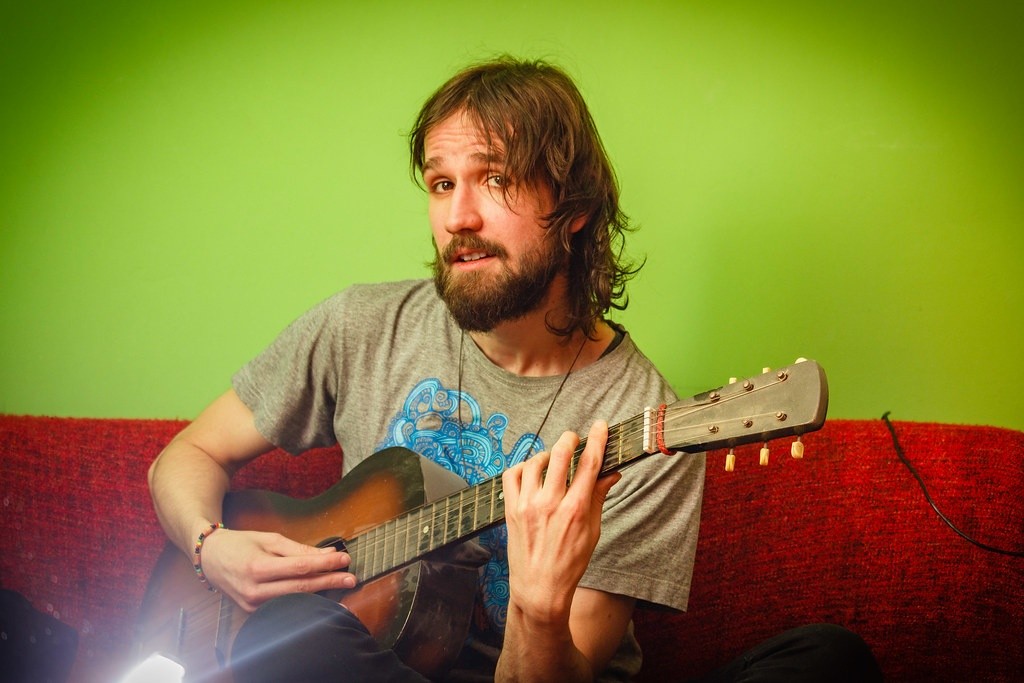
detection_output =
[147,61,706,683]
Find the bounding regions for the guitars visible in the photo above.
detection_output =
[133,355,830,682]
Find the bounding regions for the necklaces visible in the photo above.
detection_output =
[458,326,588,631]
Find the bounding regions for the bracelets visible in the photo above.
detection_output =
[193,523,224,592]
